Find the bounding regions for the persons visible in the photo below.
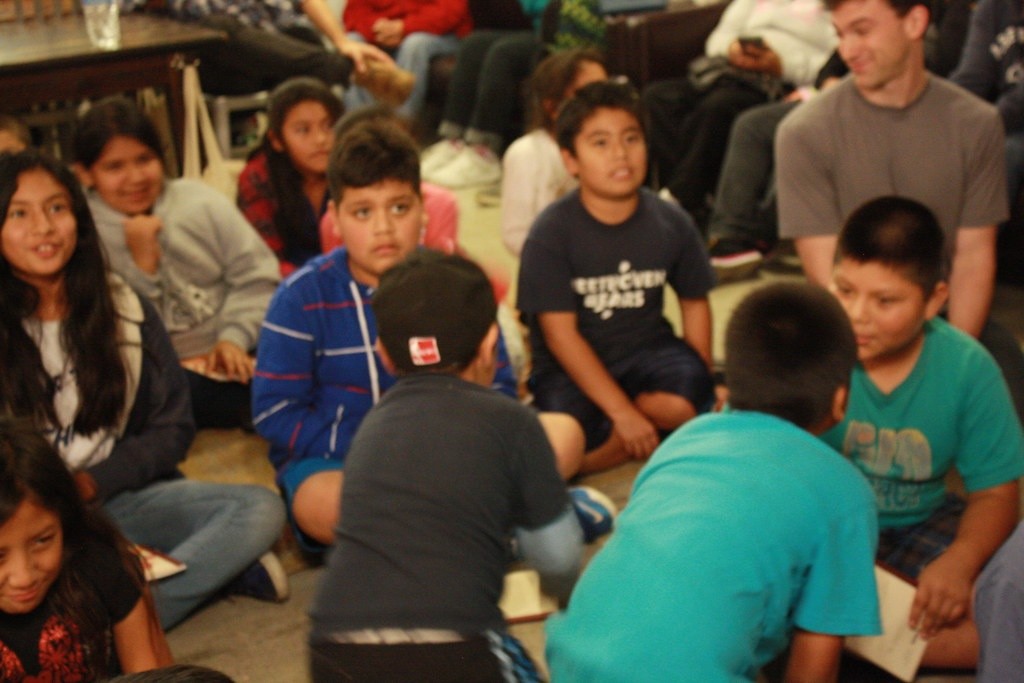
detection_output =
[0,0,1024,683]
[517,82,732,488]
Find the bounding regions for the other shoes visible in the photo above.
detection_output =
[564,484,616,534]
[707,237,762,278]
[356,56,415,106]
[419,137,503,187]
[237,550,288,602]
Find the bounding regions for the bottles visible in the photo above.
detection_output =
[80,0,122,51]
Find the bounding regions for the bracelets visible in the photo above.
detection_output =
[712,371,726,385]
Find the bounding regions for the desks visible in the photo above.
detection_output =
[0,10,227,181]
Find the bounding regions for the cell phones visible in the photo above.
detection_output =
[738,36,763,57]
